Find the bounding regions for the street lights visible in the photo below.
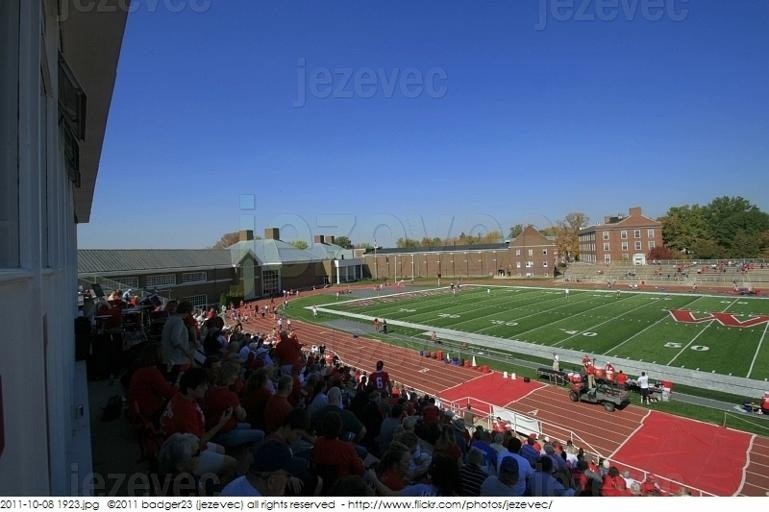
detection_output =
[373,238,378,280]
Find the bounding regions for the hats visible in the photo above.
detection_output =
[253,440,303,473]
[451,418,466,434]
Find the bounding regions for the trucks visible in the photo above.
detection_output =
[569,374,632,412]
